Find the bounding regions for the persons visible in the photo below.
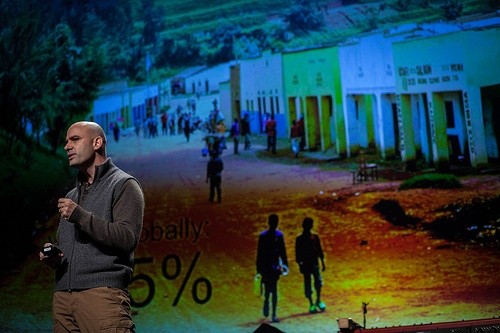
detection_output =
[254,211,291,323]
[113,103,311,158]
[293,215,329,314]
[38,120,147,332]
[204,149,224,203]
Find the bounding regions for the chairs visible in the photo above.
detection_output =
[358,148,378,180]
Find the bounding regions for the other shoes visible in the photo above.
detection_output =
[316,301,326,310]
[309,304,316,312]
[272,317,279,322]
[208,199,213,203]
